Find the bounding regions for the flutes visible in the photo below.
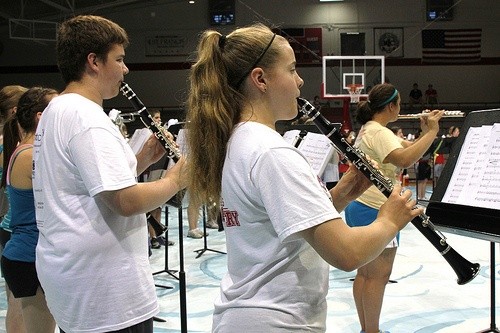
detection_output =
[120,82,182,164]
[397,110,466,119]
[297,96,482,286]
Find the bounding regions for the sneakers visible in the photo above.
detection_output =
[151,238,160,248]
[197,228,209,235]
[158,236,174,245]
[187,230,202,238]
[206,220,219,228]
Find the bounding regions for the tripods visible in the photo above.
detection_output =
[193,205,227,259]
[152,205,180,280]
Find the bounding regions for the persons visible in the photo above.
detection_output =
[415,128,431,206]
[433,126,460,178]
[0,87,59,333]
[32,15,191,333]
[178,22,424,333]
[338,133,352,180]
[146,107,175,249]
[345,83,445,333]
